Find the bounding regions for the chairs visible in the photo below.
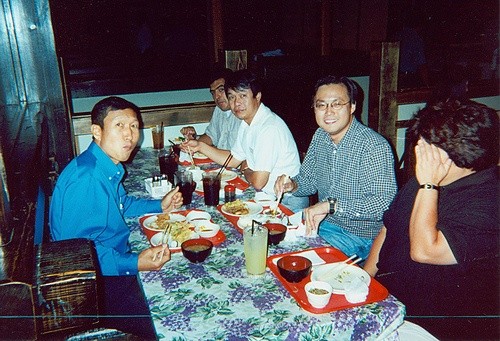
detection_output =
[0,144,125,341]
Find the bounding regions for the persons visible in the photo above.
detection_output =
[364,95,500,341]
[180,66,242,150]
[274,75,397,261]
[179,69,309,214]
[49,96,183,341]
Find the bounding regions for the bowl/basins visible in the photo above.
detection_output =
[254,192,277,207]
[180,210,222,263]
[264,224,287,246]
[145,178,173,196]
[276,256,312,283]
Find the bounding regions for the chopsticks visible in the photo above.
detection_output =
[185,138,196,167]
[336,254,364,275]
[273,174,293,211]
[153,224,174,261]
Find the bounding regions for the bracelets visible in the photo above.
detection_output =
[241,167,249,175]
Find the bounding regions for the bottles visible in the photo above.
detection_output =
[224,185,236,203]
[153,173,168,187]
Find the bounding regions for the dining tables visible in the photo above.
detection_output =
[122,141,407,341]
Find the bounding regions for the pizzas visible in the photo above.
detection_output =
[155,213,171,229]
[225,200,249,215]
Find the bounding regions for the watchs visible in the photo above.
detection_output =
[327,197,337,215]
[419,183,439,191]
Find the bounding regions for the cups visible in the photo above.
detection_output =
[158,154,221,206]
[304,279,333,309]
[152,124,182,157]
[244,225,270,274]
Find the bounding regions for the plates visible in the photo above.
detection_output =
[310,262,372,295]
[186,152,237,183]
[143,215,200,249]
[221,202,298,229]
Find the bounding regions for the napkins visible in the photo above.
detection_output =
[272,250,326,267]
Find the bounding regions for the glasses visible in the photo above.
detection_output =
[313,99,352,111]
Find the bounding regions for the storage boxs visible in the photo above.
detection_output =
[143,177,172,199]
